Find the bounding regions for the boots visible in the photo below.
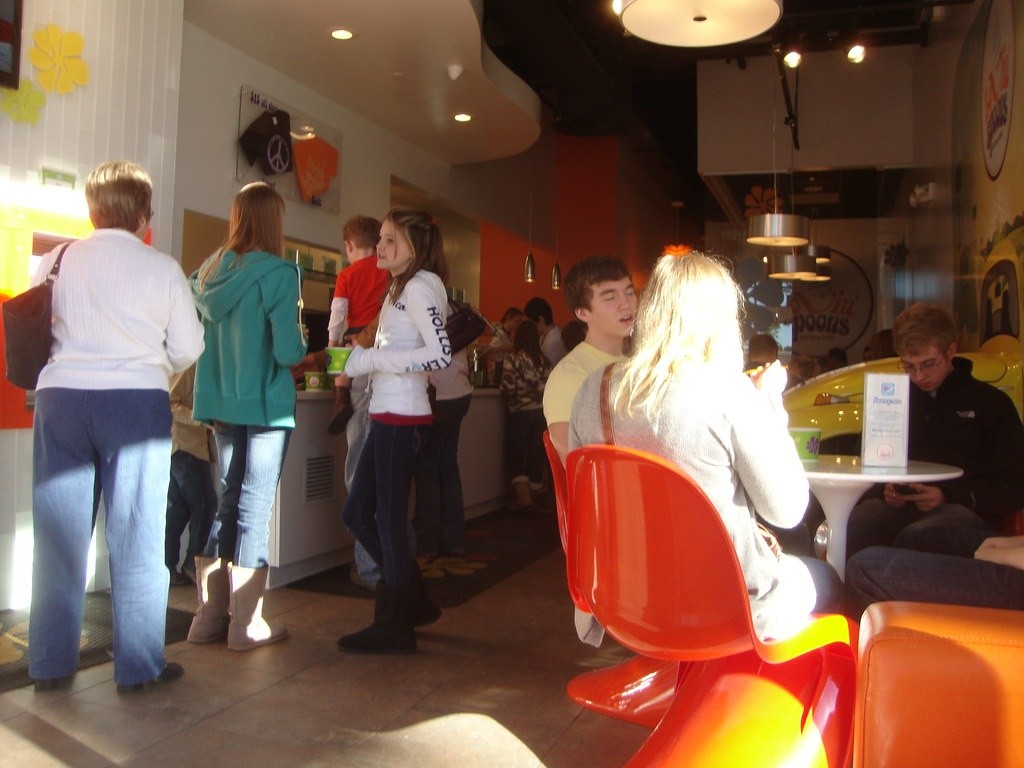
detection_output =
[227,561,287,651]
[508,475,532,511]
[186,556,234,643]
[410,560,441,626]
[336,581,416,653]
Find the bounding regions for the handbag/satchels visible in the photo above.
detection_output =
[3,243,70,388]
[444,297,485,355]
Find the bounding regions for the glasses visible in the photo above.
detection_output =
[897,350,945,374]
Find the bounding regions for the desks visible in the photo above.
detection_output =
[779,454,964,585]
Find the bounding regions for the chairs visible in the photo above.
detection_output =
[562,444,1024,768]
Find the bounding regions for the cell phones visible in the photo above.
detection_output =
[893,484,917,496]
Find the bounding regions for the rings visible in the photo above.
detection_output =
[892,492,896,500]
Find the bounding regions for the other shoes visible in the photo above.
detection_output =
[326,403,354,436]
[169,567,188,585]
[34,673,58,691]
[181,562,198,584]
[118,662,183,692]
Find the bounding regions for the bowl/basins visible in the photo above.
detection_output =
[787,428,821,463]
[304,371,326,392]
[325,346,353,374]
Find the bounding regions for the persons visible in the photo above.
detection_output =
[543,254,638,469]
[28,160,206,692]
[846,301,1024,553]
[499,320,553,512]
[413,255,466,567]
[326,215,394,435]
[292,348,329,382]
[522,296,568,498]
[863,327,899,361]
[165,361,217,586]
[188,181,309,651]
[817,347,848,373]
[845,536,1024,624]
[336,207,451,654]
[743,332,804,393]
[489,308,524,373]
[570,245,846,648]
[560,319,588,354]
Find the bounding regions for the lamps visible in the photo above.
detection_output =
[609,0,783,50]
[746,51,834,281]
[837,25,866,64]
[551,151,560,286]
[780,27,803,68]
[523,140,536,282]
